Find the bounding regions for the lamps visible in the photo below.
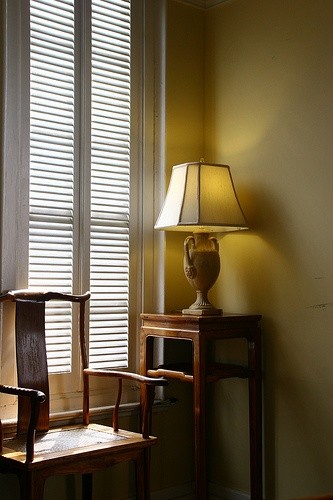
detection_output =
[153,160,251,316]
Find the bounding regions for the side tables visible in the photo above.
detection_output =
[138,312,263,500]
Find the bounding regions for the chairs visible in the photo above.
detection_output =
[0,289,168,500]
